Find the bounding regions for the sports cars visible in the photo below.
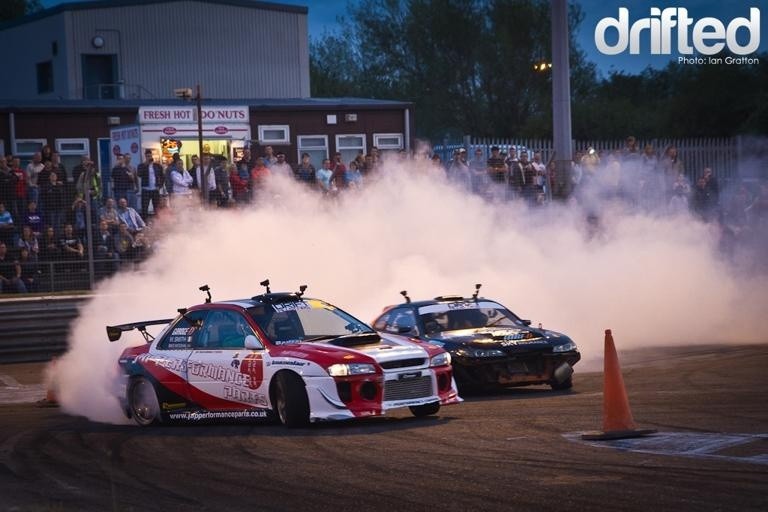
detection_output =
[374,281,582,393]
[100,280,464,426]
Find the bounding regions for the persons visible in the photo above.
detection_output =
[2,137,766,294]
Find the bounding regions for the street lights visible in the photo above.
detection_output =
[174,82,215,211]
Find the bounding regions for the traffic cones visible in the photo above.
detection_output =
[583,328,660,441]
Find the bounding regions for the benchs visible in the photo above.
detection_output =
[1,219,127,291]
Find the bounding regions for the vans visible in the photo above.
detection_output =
[436,142,546,193]
